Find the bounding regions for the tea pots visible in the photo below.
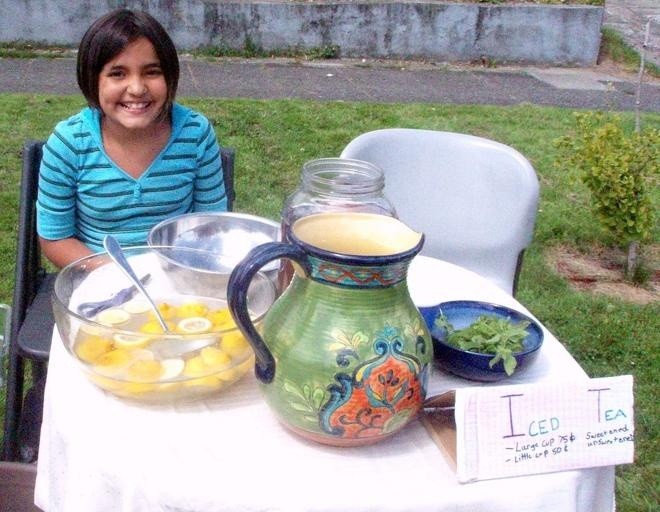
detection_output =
[225,213,435,447]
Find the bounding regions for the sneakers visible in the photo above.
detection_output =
[17,388,38,464]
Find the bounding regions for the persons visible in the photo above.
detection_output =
[15,8,230,466]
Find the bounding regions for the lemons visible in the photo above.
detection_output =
[76,302,259,395]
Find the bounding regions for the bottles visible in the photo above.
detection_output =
[281,157,398,293]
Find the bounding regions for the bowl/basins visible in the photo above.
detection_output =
[143,211,281,297]
[420,294,545,382]
[53,247,272,401]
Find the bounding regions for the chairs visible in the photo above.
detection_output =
[338,128,540,300]
[3,140,235,461]
